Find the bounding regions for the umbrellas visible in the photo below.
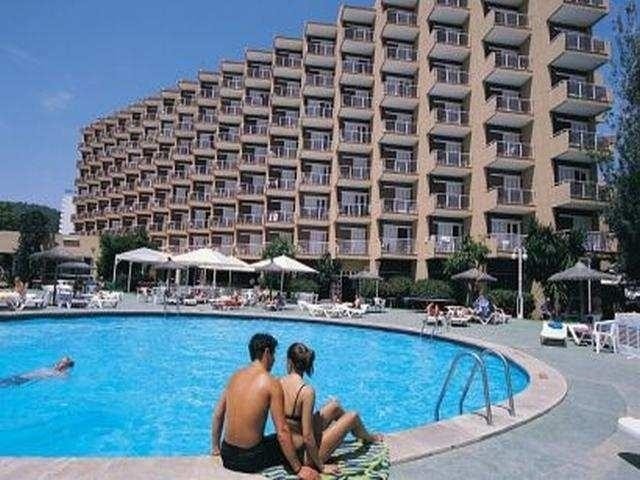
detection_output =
[29,244,97,304]
[452,267,496,305]
[349,270,384,282]
[548,261,617,318]
[174,245,252,288]
[250,253,321,292]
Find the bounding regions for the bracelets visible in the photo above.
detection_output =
[295,466,302,475]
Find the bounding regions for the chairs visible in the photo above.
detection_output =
[0,285,125,311]
[540,316,619,355]
[137,284,385,320]
[427,305,513,329]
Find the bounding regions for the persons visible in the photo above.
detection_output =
[427,302,440,317]
[6,276,283,322]
[212,332,321,480]
[352,294,361,310]
[280,342,382,477]
[0,357,74,391]
